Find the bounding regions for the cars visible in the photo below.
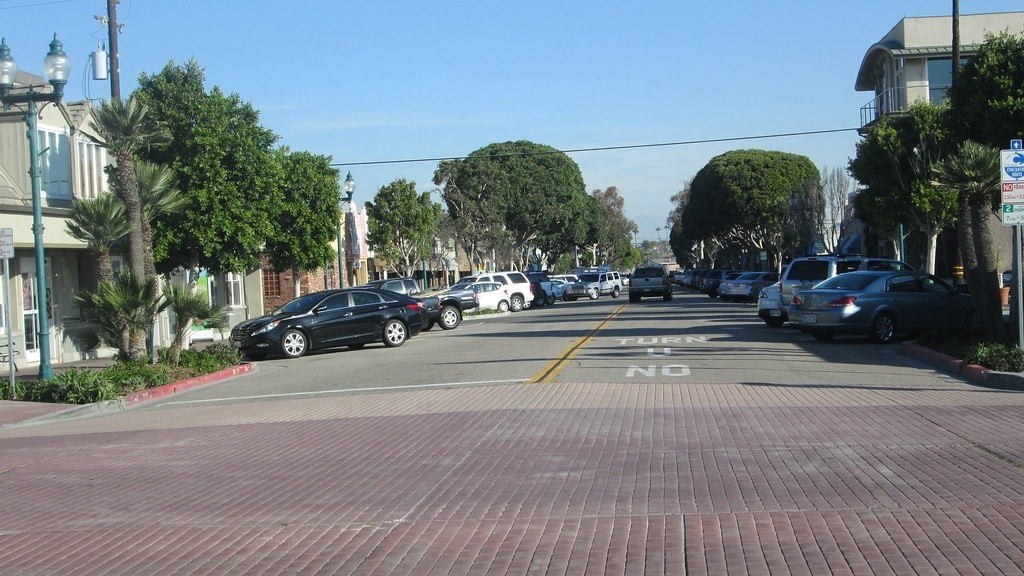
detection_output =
[357,277,481,332]
[228,286,423,359]
[757,279,789,328]
[522,263,629,310]
[665,267,747,300]
[786,268,994,345]
[724,271,782,301]
[442,281,512,315]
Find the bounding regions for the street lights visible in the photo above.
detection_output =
[655,227,662,259]
[664,226,670,261]
[0,31,72,378]
[334,169,357,288]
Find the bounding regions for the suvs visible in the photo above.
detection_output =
[627,265,676,302]
[777,253,922,315]
[565,269,622,300]
[457,270,535,312]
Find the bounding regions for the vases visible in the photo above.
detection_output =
[999,286,1011,304]
